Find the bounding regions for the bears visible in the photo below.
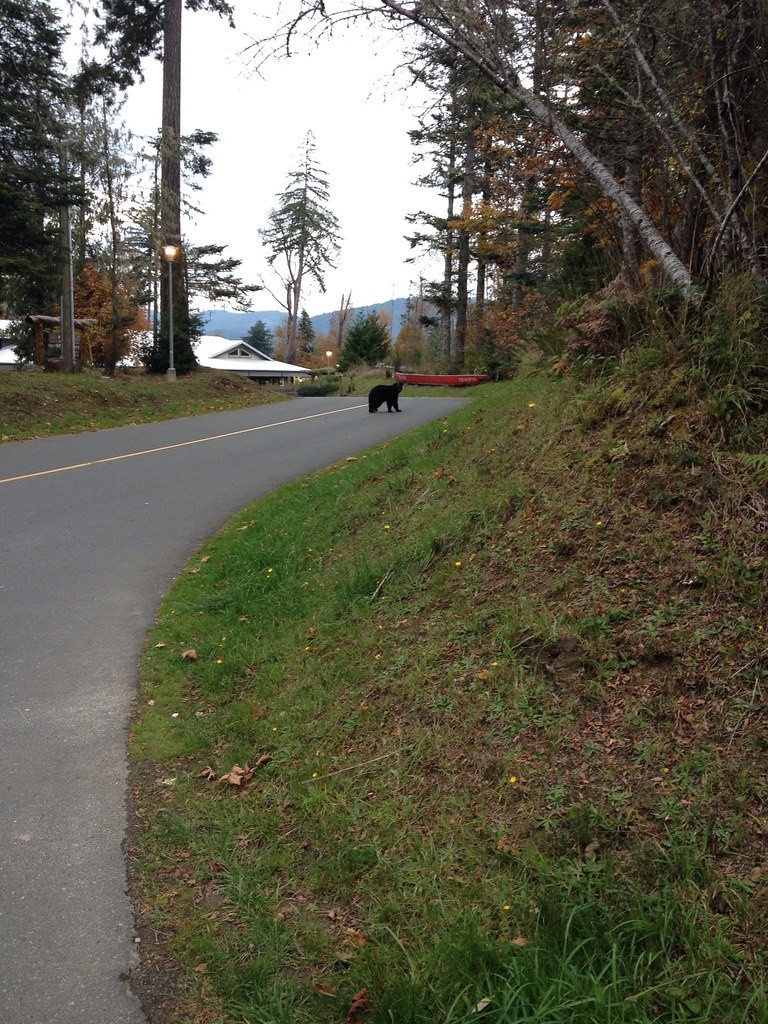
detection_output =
[369,382,403,413]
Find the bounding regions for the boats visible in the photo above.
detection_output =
[394,372,491,386]
[161,244,182,382]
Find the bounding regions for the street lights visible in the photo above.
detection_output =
[326,350,334,375]
